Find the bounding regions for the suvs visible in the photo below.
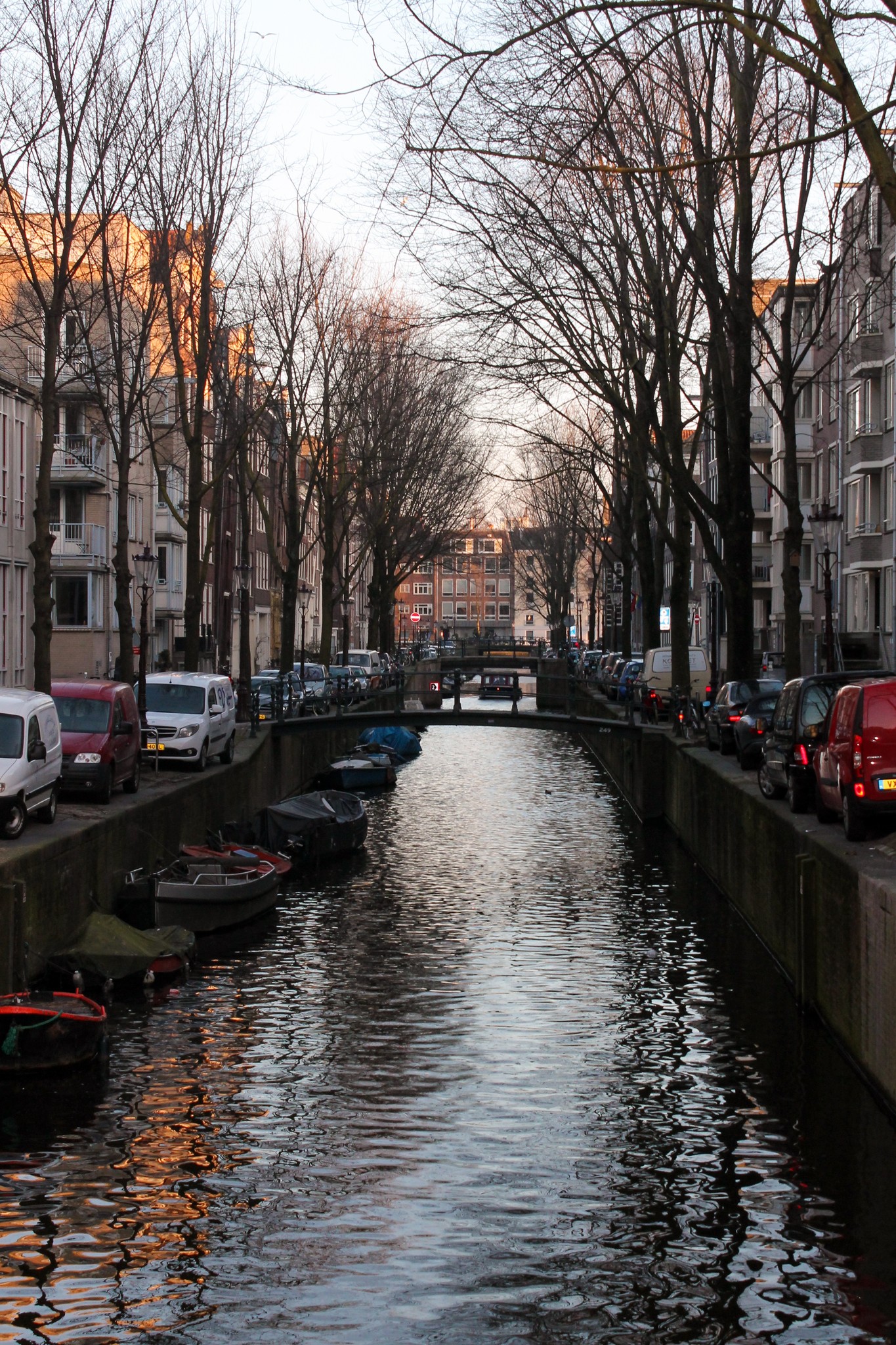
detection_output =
[702,670,896,841]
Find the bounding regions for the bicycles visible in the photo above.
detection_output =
[388,651,410,667]
[667,679,698,739]
[631,676,661,725]
[332,678,353,708]
[305,681,329,717]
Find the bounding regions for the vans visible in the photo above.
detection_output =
[133,672,235,773]
[632,647,712,711]
[0,687,63,839]
[33,680,141,805]
[333,649,381,691]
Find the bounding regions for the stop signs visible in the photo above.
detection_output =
[695,614,699,624]
[410,612,421,623]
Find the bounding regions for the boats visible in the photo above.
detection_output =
[0,971,106,1080]
[478,671,522,700]
[265,790,367,857]
[47,911,195,995]
[316,722,429,794]
[125,844,292,906]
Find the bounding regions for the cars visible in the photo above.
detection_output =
[237,662,369,719]
[547,637,644,703]
[378,641,455,680]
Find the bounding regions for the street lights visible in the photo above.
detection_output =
[607,586,623,652]
[688,596,701,645]
[297,582,313,717]
[130,542,161,770]
[702,556,722,713]
[396,597,406,663]
[433,620,439,645]
[426,619,430,639]
[451,611,457,635]
[442,620,448,648]
[806,496,843,685]
[575,598,584,646]
[411,620,415,645]
[402,615,408,648]
